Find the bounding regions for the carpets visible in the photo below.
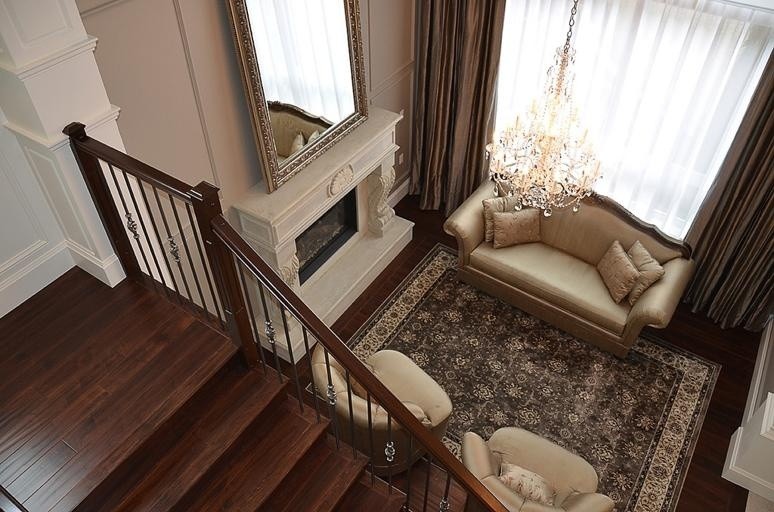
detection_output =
[304,241,722,511]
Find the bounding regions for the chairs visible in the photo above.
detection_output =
[461,424,616,511]
[307,337,454,477]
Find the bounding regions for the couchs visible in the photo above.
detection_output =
[267,100,334,167]
[443,173,696,358]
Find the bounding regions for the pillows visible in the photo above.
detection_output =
[479,196,541,249]
[597,240,666,305]
[290,130,321,155]
[499,460,559,506]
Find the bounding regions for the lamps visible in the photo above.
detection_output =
[482,0,609,216]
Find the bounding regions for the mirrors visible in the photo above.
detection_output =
[224,0,370,194]
